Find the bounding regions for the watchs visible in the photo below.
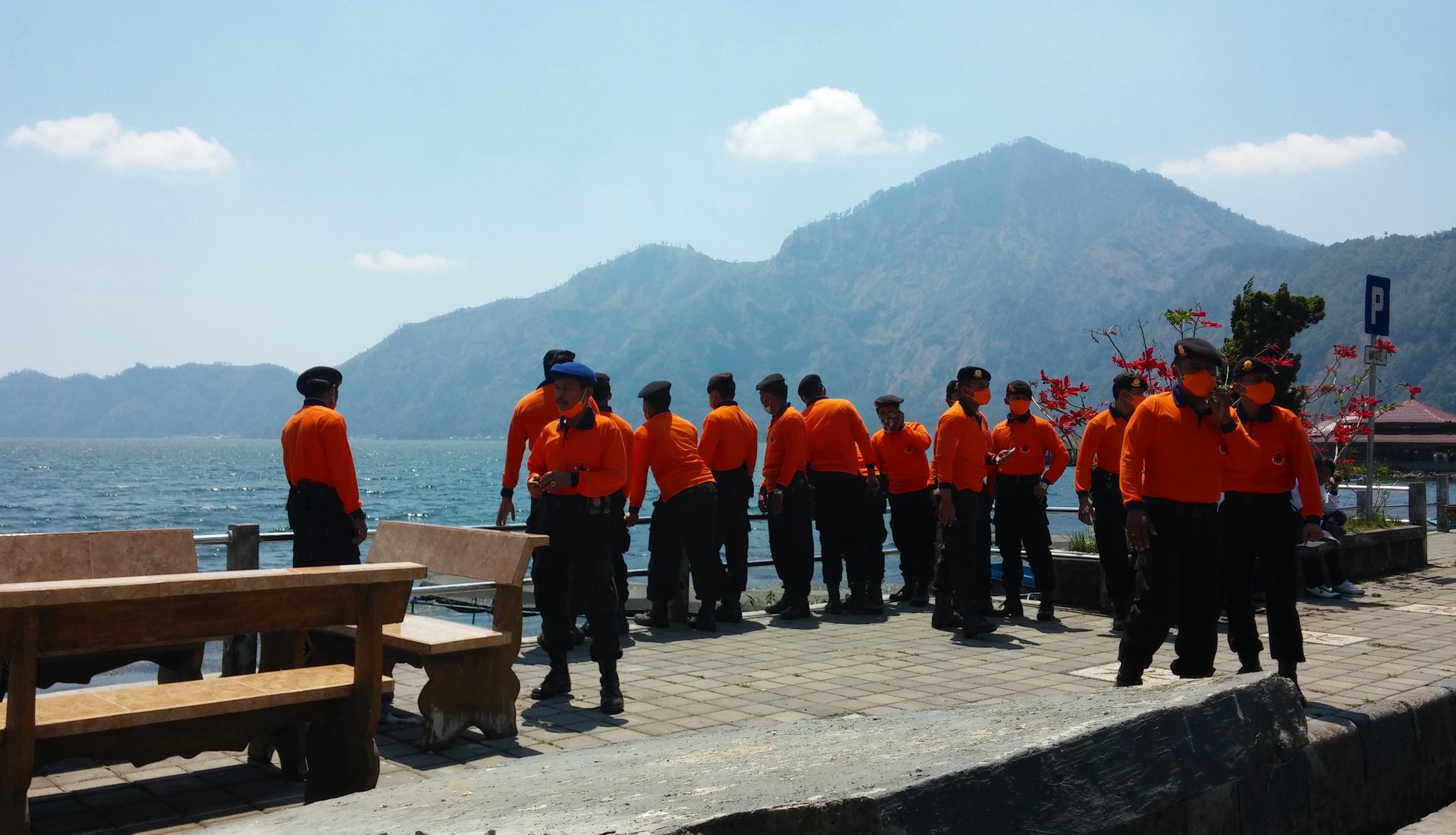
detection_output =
[774,489,783,494]
[353,509,366,519]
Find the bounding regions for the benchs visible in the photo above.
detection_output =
[310,520,549,749]
[0,560,428,835]
[0,529,209,705]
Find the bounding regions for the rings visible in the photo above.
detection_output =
[1131,534,1136,536]
[363,535,366,538]
[551,480,557,488]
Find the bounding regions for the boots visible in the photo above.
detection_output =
[599,679,624,713]
[996,600,1023,617]
[1112,594,1307,707]
[1037,597,1054,620]
[531,663,571,699]
[536,574,998,650]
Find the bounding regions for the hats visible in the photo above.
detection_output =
[1233,357,1274,378]
[543,349,575,366]
[957,366,991,381]
[707,372,733,394]
[1174,337,1223,367]
[550,362,599,389]
[638,380,671,398]
[1113,373,1148,393]
[874,394,904,408]
[1006,380,1032,400]
[798,374,823,396]
[946,380,959,393]
[593,372,610,384]
[297,366,342,395]
[756,374,785,391]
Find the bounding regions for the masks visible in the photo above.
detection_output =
[960,386,991,405]
[763,393,771,414]
[330,386,336,410]
[558,387,588,419]
[644,402,650,420]
[1007,397,1030,415]
[1237,380,1275,405]
[1121,389,1146,410]
[709,391,716,409]
[1176,365,1217,397]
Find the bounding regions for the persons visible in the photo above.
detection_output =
[578,367,634,640]
[795,365,1368,640]
[1216,356,1325,711]
[526,361,629,714]
[756,374,816,621]
[685,370,758,631]
[280,365,368,568]
[1114,338,1263,691]
[623,381,729,633]
[495,348,602,654]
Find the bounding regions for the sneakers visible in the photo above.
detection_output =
[1296,540,1336,558]
[1332,579,1365,595]
[1319,584,1343,598]
[1306,587,1337,599]
[1320,529,1341,550]
